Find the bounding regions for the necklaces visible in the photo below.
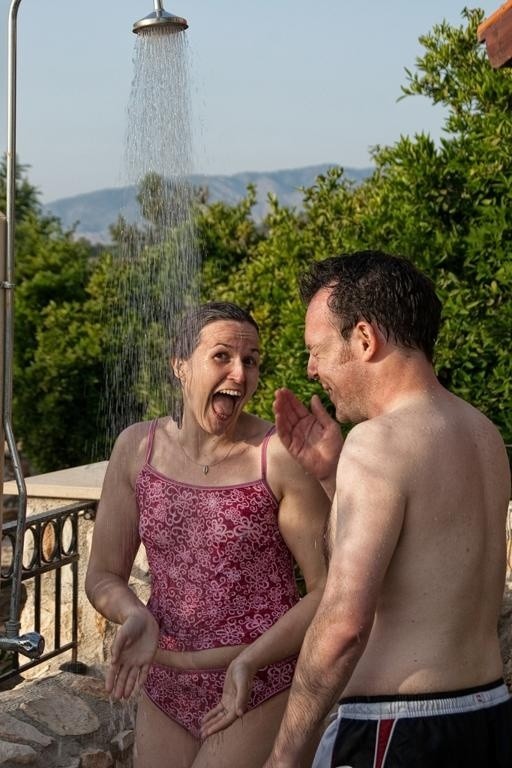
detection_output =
[171,423,242,479]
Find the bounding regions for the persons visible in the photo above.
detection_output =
[85,300,335,767]
[262,249,510,768]
